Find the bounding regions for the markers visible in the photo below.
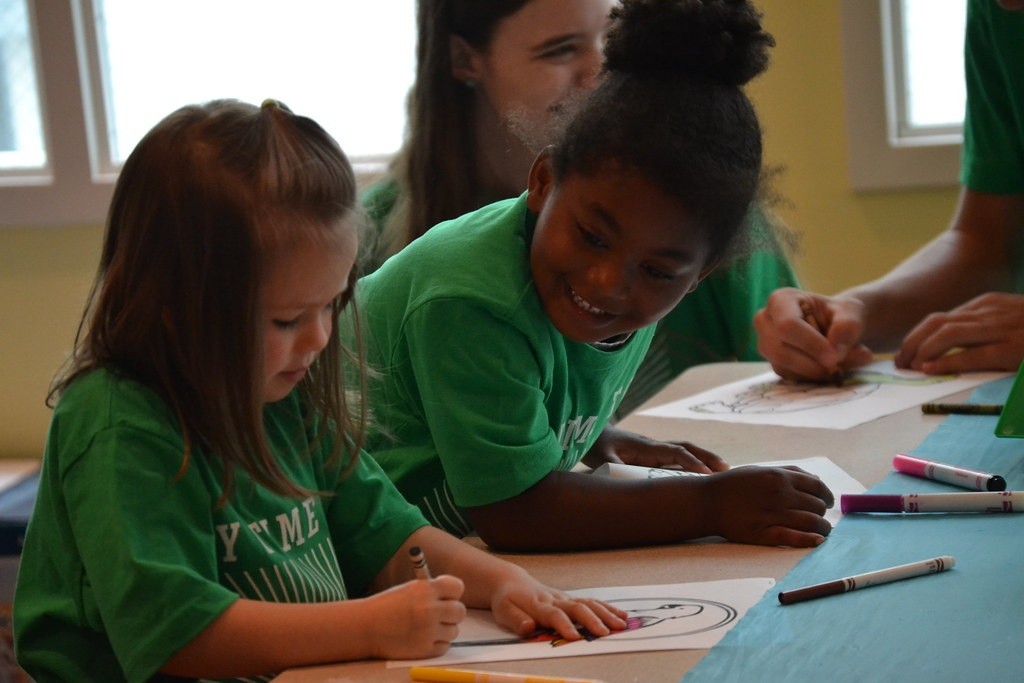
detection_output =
[840,490,1024,514]
[892,454,1007,492]
[778,554,956,605]
[921,401,1003,416]
[799,300,843,389]
[410,666,601,683]
[409,546,433,579]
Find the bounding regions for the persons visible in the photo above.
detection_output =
[363,1,799,408]
[757,0,1023,384]
[334,1,835,553]
[12,97,632,683]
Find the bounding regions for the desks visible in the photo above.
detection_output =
[268,360,1024,683]
